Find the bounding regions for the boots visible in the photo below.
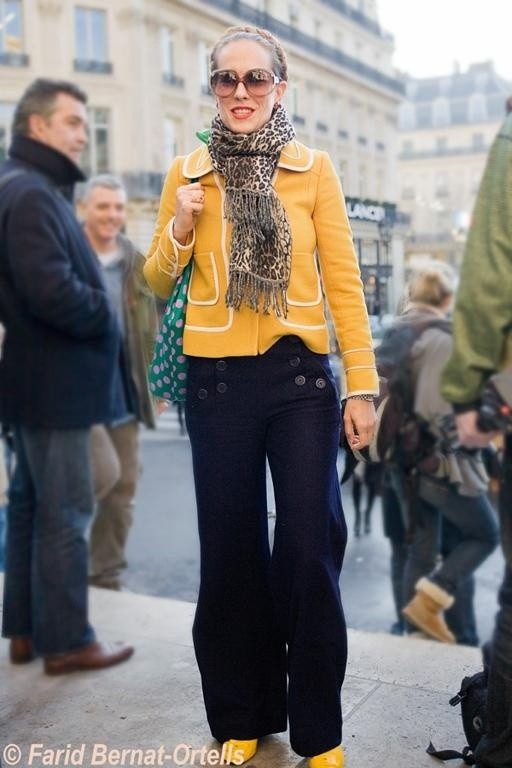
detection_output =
[401,577,456,643]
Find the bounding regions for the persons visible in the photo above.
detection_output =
[142,22,383,768]
[76,168,159,593]
[1,75,137,676]
[338,254,499,648]
[436,88,511,768]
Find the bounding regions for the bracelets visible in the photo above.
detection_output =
[348,394,373,401]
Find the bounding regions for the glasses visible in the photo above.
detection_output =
[210,68,280,98]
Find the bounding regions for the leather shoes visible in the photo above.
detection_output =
[222,739,258,766]
[9,636,134,677]
[307,746,344,768]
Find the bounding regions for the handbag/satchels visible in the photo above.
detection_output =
[426,671,490,766]
[147,263,192,402]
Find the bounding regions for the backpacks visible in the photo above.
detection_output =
[339,318,454,495]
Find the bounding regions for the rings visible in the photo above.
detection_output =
[199,196,202,203]
